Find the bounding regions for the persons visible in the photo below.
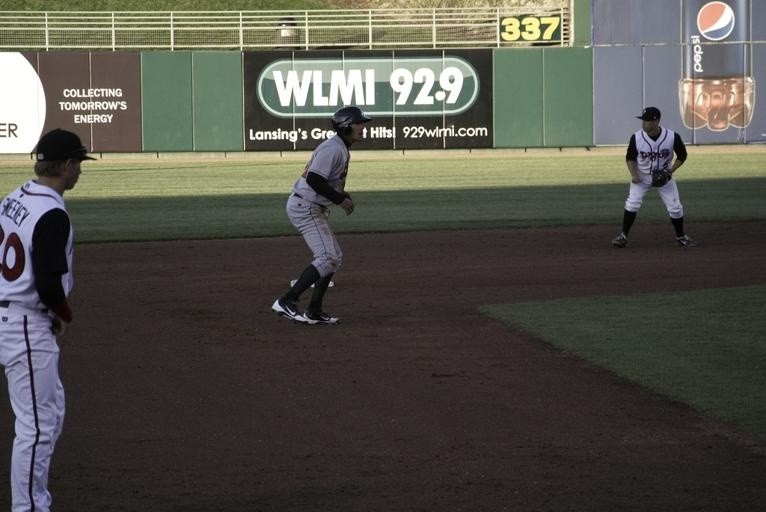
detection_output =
[680,77,754,130]
[611,107,694,247]
[1,130,97,511]
[270,106,372,324]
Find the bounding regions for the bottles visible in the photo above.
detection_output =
[677,1,757,132]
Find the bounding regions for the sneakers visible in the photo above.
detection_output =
[612,232,628,246]
[675,235,697,248]
[271,296,309,324]
[302,308,341,326]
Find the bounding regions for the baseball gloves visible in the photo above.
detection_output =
[651,168,672,188]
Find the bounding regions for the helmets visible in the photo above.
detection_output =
[331,106,373,134]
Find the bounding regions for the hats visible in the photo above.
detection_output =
[637,106,662,122]
[35,128,98,163]
